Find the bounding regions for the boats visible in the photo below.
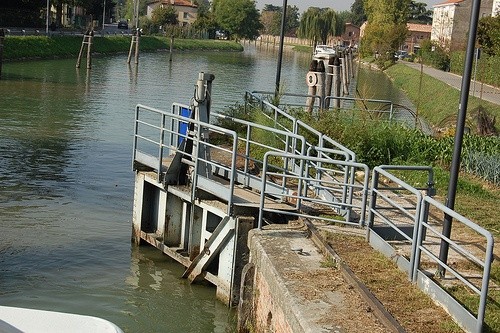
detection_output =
[313,44,337,60]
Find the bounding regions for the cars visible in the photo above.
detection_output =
[118,20,128,29]
